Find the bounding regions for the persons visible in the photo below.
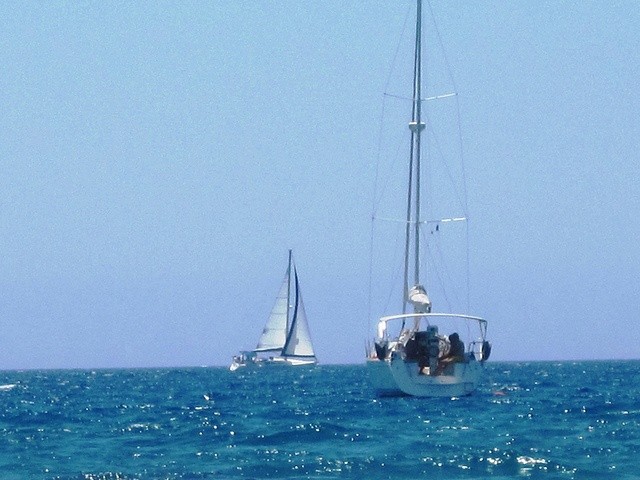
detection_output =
[449,333,465,357]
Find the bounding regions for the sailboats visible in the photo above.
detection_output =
[230,249,319,372]
[365,1,491,397]
